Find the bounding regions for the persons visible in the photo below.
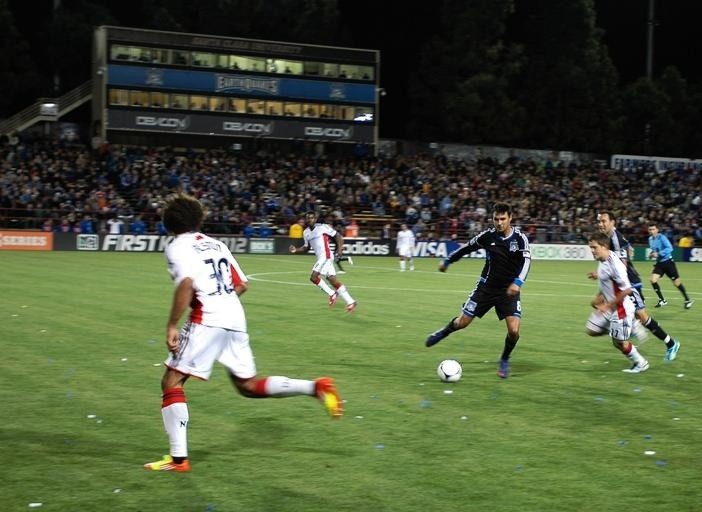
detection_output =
[288,211,357,318]
[584,234,649,374]
[144,193,343,472]
[590,211,680,360]
[424,203,531,379]
[647,224,695,309]
[0,49,702,274]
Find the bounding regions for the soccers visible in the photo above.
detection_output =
[436,359,463,383]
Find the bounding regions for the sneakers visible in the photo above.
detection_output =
[622,360,649,372]
[144,455,190,471]
[426,330,443,346]
[315,377,342,418]
[684,298,690,308]
[655,299,667,308]
[666,339,680,360]
[337,270,345,274]
[347,257,353,264]
[497,359,508,378]
[345,302,356,312]
[329,292,338,305]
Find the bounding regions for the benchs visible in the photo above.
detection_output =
[0,191,577,244]
